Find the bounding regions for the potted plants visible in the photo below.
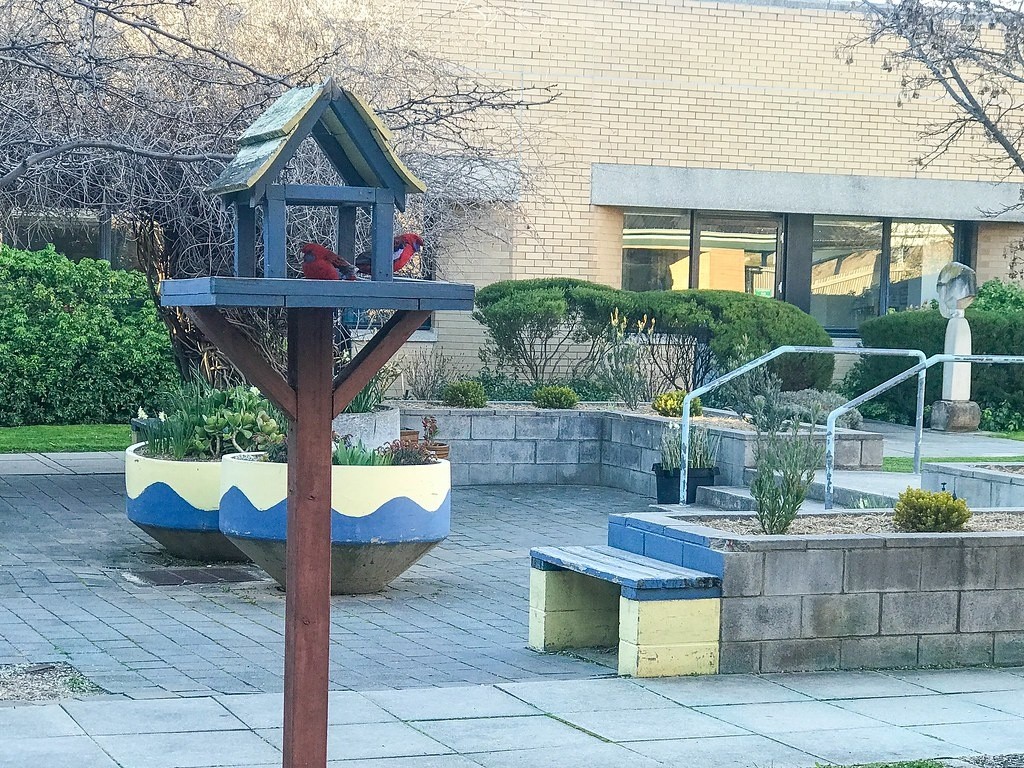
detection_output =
[418,415,450,460]
[650,420,723,505]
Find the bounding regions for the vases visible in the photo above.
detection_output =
[399,426,421,447]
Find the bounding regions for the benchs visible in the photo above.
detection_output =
[527,542,723,678]
